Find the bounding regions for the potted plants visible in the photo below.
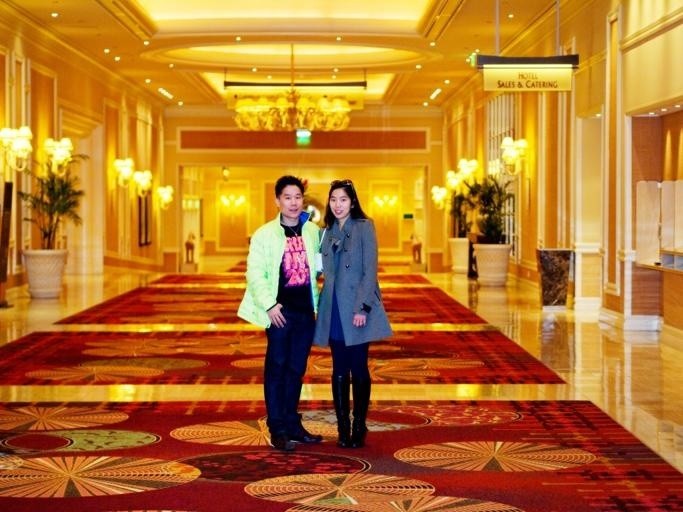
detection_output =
[462,176,513,287]
[447,193,474,272]
[20,153,91,299]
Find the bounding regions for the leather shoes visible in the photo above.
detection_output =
[271,431,323,450]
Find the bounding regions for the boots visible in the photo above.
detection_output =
[351,377,371,448]
[332,376,350,448]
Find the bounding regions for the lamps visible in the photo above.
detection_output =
[114,158,135,187]
[160,185,175,205]
[226,42,354,132]
[0,126,33,170]
[132,170,154,197]
[430,137,527,210]
[45,136,74,164]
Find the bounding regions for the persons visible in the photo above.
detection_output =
[312,179,393,449]
[235,174,323,452]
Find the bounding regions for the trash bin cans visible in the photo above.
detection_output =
[535,248,571,306]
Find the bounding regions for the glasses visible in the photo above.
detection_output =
[330,180,353,190]
